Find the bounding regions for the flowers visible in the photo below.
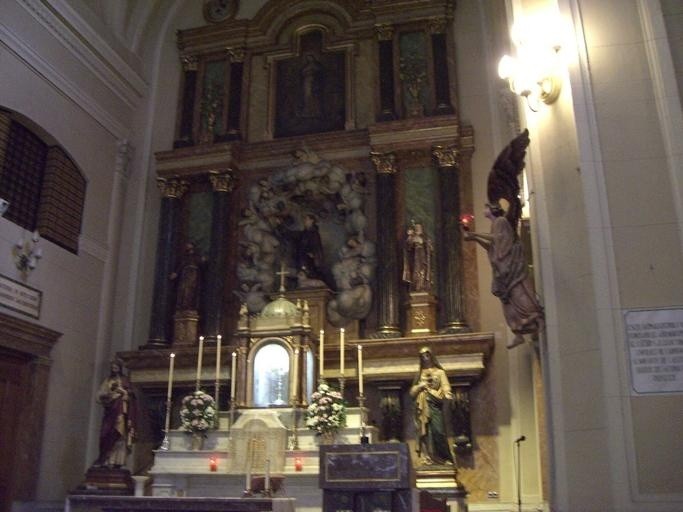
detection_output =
[302,381,349,438]
[180,391,219,439]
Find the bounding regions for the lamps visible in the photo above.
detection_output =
[496,0,565,114]
[11,228,44,282]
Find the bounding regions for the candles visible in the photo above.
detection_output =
[294,459,302,471]
[207,459,217,472]
[166,334,238,401]
[292,326,364,398]
[246,459,269,489]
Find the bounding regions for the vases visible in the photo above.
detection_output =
[321,432,336,445]
[191,431,203,451]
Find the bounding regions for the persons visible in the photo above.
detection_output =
[460,201,546,351]
[408,346,460,467]
[88,357,137,471]
[167,236,209,312]
[298,50,331,118]
[401,221,433,291]
[230,143,377,325]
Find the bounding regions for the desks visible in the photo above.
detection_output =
[65,495,297,512]
